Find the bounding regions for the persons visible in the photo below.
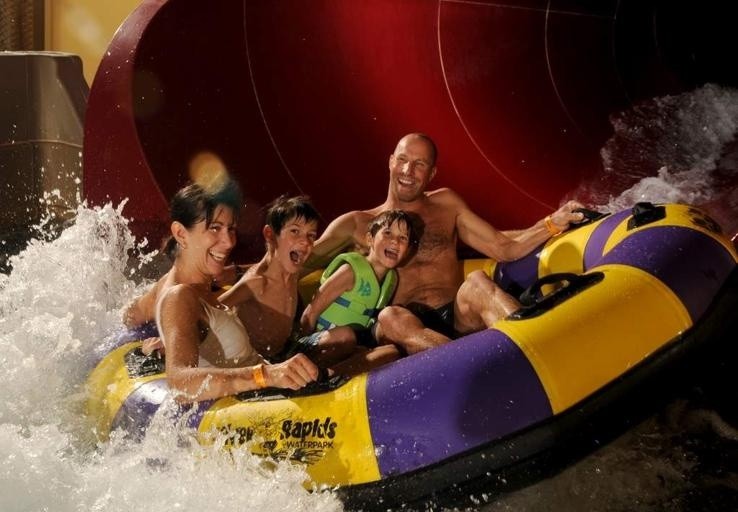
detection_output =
[210,133,586,356]
[297,210,413,381]
[217,196,358,396]
[122,178,318,405]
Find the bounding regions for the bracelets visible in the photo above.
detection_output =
[543,214,561,237]
[251,364,267,390]
[234,262,241,281]
